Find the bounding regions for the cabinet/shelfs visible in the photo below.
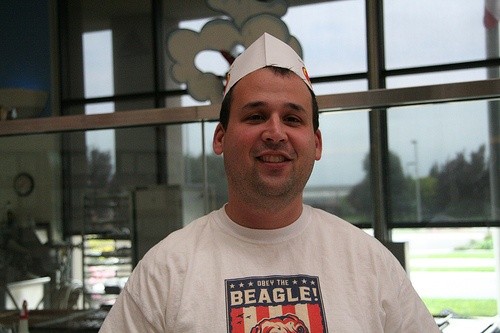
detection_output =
[81,185,181,315]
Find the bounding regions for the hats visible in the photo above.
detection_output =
[224,32,316,101]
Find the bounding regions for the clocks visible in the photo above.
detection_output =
[14,172,34,197]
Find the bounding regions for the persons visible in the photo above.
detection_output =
[98,32,442,333]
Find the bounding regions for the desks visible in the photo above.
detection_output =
[1,276,50,315]
